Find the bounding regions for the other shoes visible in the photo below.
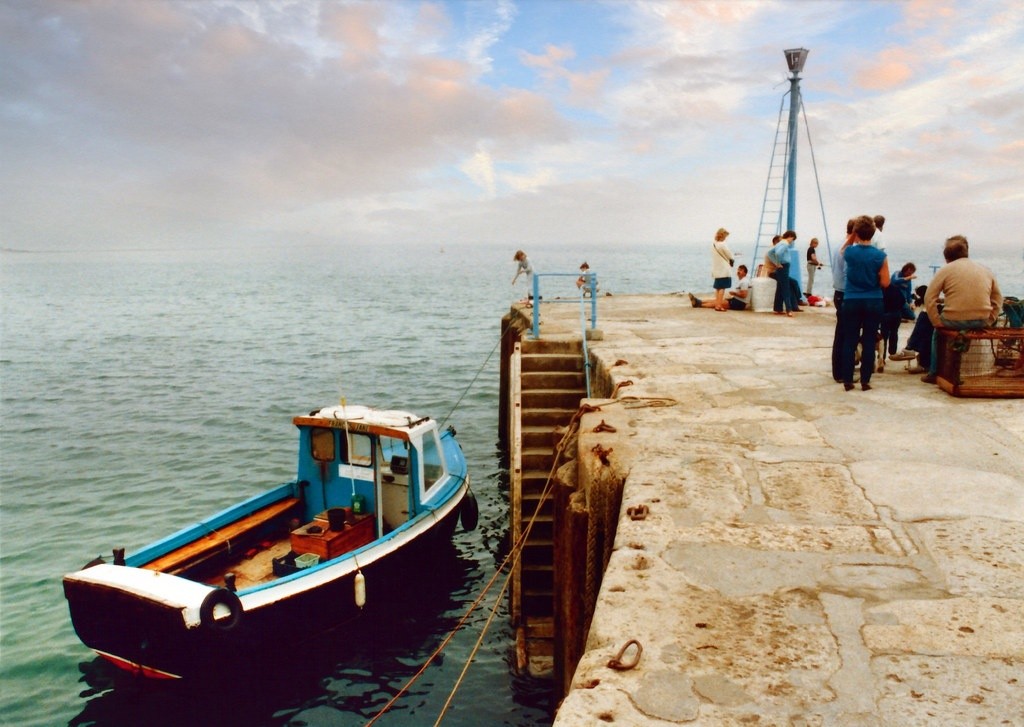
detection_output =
[862,383,871,391]
[793,308,804,312]
[921,375,937,383]
[689,293,702,307]
[787,311,792,316]
[798,300,808,306]
[878,364,884,373]
[844,382,854,391]
[773,311,785,314]
[837,379,844,383]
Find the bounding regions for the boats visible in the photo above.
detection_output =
[61,399,479,702]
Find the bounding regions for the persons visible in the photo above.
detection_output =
[712,228,734,312]
[512,250,542,301]
[870,216,886,253]
[806,238,822,294]
[888,236,968,375]
[831,220,861,384]
[764,235,808,312]
[890,263,916,324]
[577,263,598,298]
[921,240,1004,385]
[689,265,753,311]
[767,230,796,317]
[841,216,890,391]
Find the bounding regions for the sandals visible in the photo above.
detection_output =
[715,307,727,311]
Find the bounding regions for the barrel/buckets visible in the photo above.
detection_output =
[752,277,777,313]
[352,494,365,514]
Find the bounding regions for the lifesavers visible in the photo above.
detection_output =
[199,587,245,638]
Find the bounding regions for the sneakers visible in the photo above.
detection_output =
[889,347,915,360]
[908,366,926,375]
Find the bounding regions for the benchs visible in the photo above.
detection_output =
[138,496,300,575]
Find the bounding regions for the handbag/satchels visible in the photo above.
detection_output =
[1003,297,1024,327]
[730,259,734,267]
